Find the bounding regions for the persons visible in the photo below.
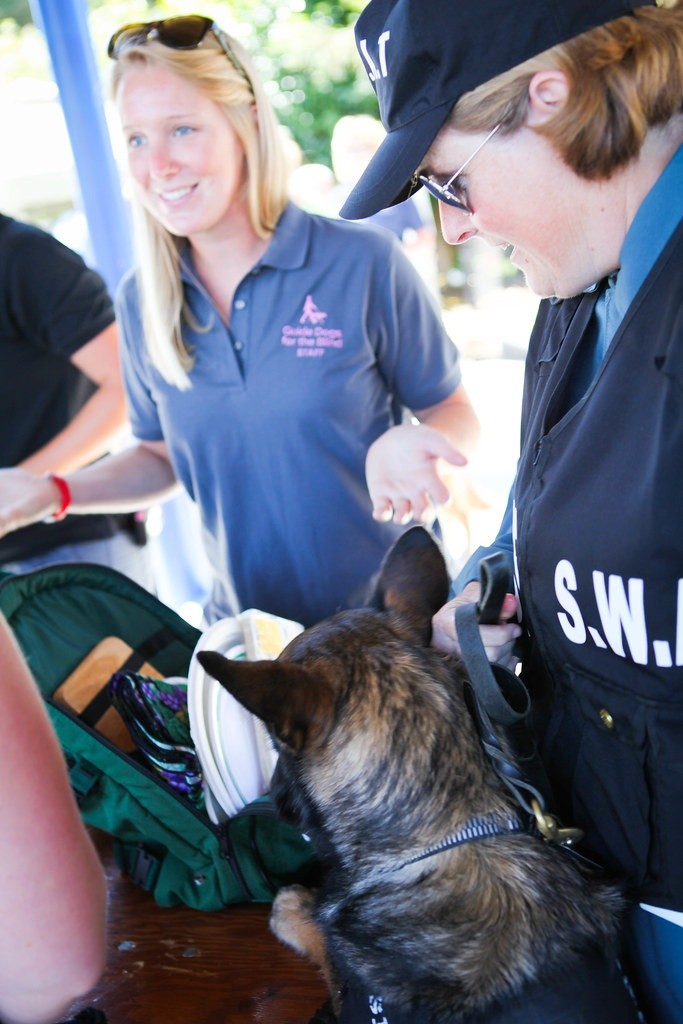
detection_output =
[0,0,683,1024]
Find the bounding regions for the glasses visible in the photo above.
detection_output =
[415,93,529,214]
[107,15,257,110]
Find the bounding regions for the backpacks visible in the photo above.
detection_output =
[0,561,319,912]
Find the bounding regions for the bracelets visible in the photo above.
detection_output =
[46,472,72,523]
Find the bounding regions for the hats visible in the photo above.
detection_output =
[337,0,649,219]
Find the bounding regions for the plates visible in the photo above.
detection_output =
[188,616,267,826]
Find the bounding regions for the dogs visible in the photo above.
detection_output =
[196,525,647,1024]
